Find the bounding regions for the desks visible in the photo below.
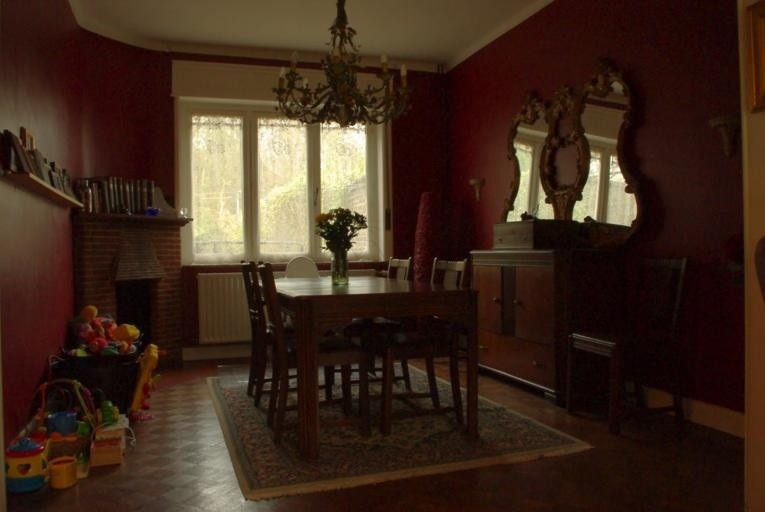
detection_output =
[255,274,481,465]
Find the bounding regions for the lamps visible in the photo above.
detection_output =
[270,1,414,131]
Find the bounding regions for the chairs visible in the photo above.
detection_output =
[240,251,471,446]
[561,254,690,432]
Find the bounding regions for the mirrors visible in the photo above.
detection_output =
[493,51,647,248]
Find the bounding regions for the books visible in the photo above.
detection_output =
[2,129,155,215]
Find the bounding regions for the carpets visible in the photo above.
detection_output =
[203,360,601,503]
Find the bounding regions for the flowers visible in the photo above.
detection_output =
[313,208,369,282]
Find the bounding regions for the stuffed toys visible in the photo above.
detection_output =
[64,305,141,357]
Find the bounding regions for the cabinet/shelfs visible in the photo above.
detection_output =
[466,246,623,409]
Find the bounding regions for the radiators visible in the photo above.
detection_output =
[195,271,252,345]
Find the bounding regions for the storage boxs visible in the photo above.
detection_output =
[493,220,547,248]
